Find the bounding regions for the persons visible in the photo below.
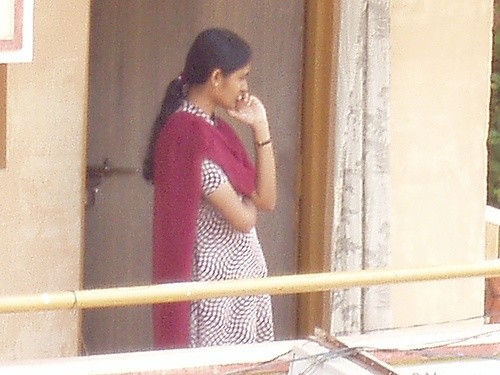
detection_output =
[140,26,280,350]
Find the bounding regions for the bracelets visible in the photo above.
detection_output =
[254,136,273,146]
